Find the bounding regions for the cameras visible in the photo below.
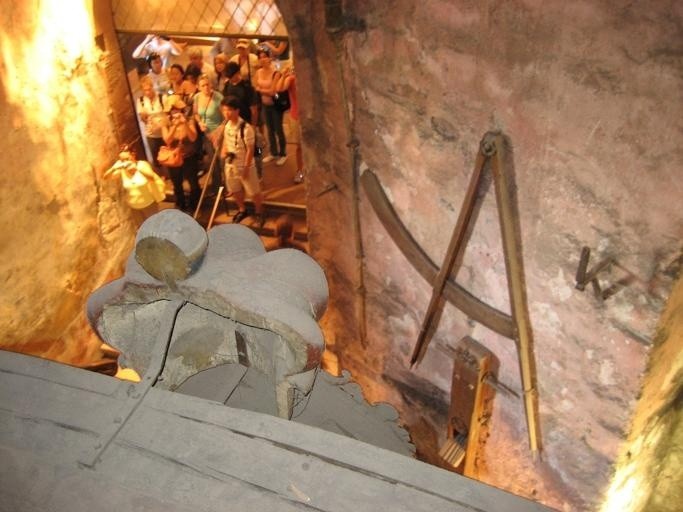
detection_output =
[121,161,129,168]
[167,89,175,95]
[227,152,235,164]
[172,114,180,119]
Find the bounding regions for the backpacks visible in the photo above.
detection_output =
[240,122,264,171]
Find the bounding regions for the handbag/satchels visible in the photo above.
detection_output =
[137,56,149,76]
[146,172,167,202]
[158,146,184,168]
[193,121,204,159]
[272,90,291,112]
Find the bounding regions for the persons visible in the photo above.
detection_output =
[132,32,304,228]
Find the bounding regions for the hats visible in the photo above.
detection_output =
[163,95,186,112]
[236,39,252,49]
[224,62,239,78]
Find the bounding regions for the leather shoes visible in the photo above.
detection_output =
[233,210,264,225]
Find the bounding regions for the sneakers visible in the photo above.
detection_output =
[294,170,303,182]
[203,188,232,197]
[262,154,287,165]
